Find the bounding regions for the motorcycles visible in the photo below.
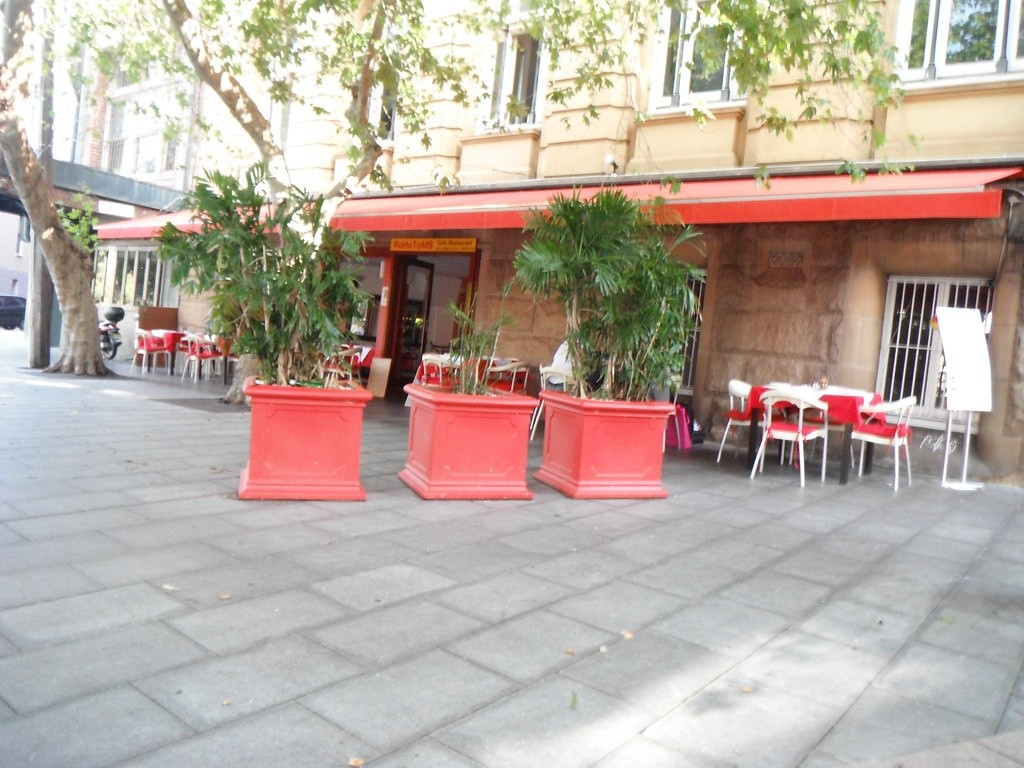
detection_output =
[98,307,125,359]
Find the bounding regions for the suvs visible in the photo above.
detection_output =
[0,296,26,330]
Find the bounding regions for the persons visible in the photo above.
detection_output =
[548,338,574,392]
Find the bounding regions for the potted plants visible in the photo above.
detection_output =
[397,275,540,501]
[150,160,375,502]
[512,179,707,500]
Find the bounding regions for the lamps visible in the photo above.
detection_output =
[604,154,618,173]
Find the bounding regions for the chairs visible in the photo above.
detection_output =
[663,376,682,453]
[715,377,918,493]
[129,327,241,384]
[323,347,363,388]
[422,356,530,397]
[529,368,576,441]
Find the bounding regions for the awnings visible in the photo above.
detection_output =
[97,203,281,239]
[329,166,1024,231]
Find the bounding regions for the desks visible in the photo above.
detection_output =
[404,353,528,406]
[147,329,190,376]
[179,333,232,385]
[749,384,887,487]
